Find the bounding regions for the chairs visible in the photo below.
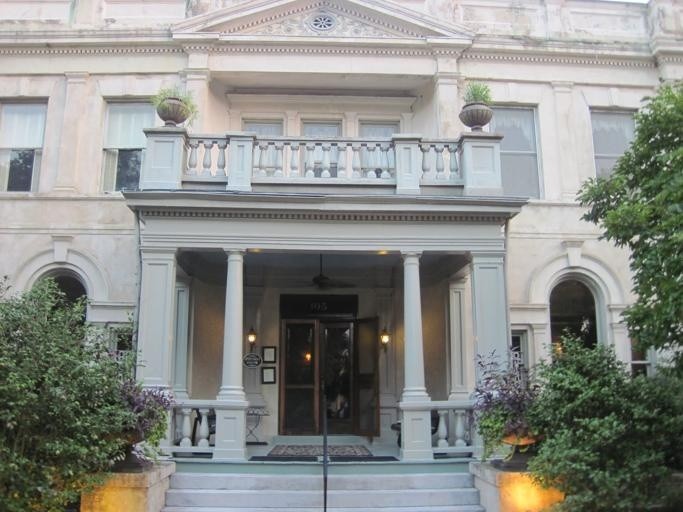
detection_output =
[188,403,215,447]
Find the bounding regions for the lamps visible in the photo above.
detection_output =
[312,253,329,289]
[246,324,256,352]
[378,325,389,354]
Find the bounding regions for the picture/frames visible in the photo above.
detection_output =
[260,367,275,384]
[261,346,276,363]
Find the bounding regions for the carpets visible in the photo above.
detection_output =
[266,444,372,460]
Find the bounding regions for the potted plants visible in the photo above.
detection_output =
[461,346,567,512]
[79,375,176,512]
[148,84,199,130]
[458,78,494,134]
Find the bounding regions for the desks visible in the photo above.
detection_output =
[245,411,270,444]
